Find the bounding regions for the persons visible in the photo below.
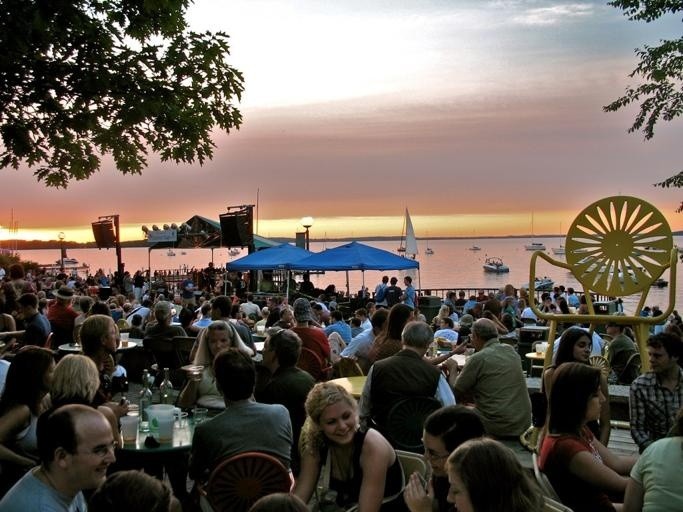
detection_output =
[1,260,682,511]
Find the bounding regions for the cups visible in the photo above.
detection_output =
[463,347,475,365]
[173,412,189,430]
[191,407,209,429]
[190,366,204,382]
[257,326,266,336]
[119,332,130,347]
[535,343,547,359]
[119,415,142,445]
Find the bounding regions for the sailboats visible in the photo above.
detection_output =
[524,207,546,250]
[396,208,434,271]
[551,222,589,254]
[468,228,482,251]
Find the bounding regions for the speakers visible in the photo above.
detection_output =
[220,215,251,247]
[92,220,117,248]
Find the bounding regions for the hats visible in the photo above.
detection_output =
[51,285,78,299]
[293,298,312,322]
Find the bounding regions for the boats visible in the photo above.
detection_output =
[55,257,78,264]
[227,249,241,256]
[522,277,555,290]
[165,248,176,256]
[482,255,510,274]
[569,258,668,287]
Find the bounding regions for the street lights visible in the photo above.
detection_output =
[57,231,65,269]
[301,217,313,283]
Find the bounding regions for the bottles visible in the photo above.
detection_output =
[138,369,153,433]
[159,367,175,404]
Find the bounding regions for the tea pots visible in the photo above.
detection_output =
[142,402,183,444]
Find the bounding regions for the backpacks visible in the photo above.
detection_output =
[375,285,385,303]
